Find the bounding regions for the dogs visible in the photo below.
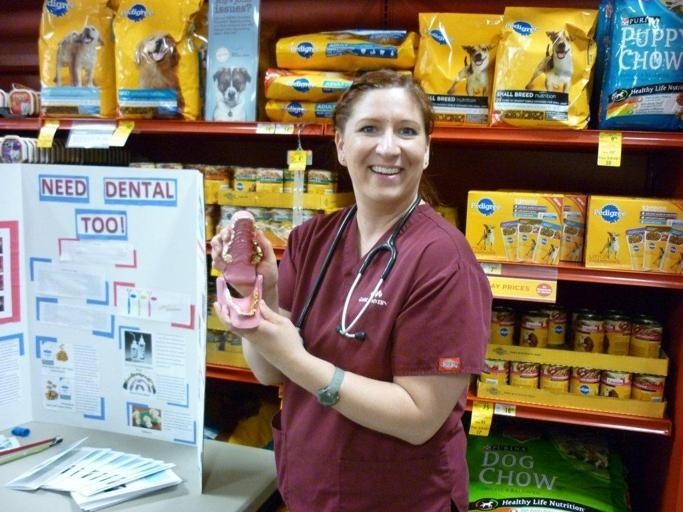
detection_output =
[447,42,498,101]
[477,223,500,255]
[211,65,254,123]
[53,26,101,90]
[525,28,579,101]
[132,31,187,120]
[601,231,624,263]
[567,240,587,263]
[542,244,561,268]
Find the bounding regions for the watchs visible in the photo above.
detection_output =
[316,366,344,406]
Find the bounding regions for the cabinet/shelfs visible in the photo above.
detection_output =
[1,0,682,511]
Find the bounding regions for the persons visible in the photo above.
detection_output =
[210,72,493,512]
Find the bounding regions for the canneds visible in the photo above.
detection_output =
[489,310,515,344]
[543,308,569,345]
[521,309,552,345]
[602,369,635,397]
[576,312,603,353]
[510,363,537,389]
[604,314,630,355]
[635,373,666,401]
[481,360,507,385]
[633,320,662,358]
[571,367,603,397]
[541,364,567,392]
